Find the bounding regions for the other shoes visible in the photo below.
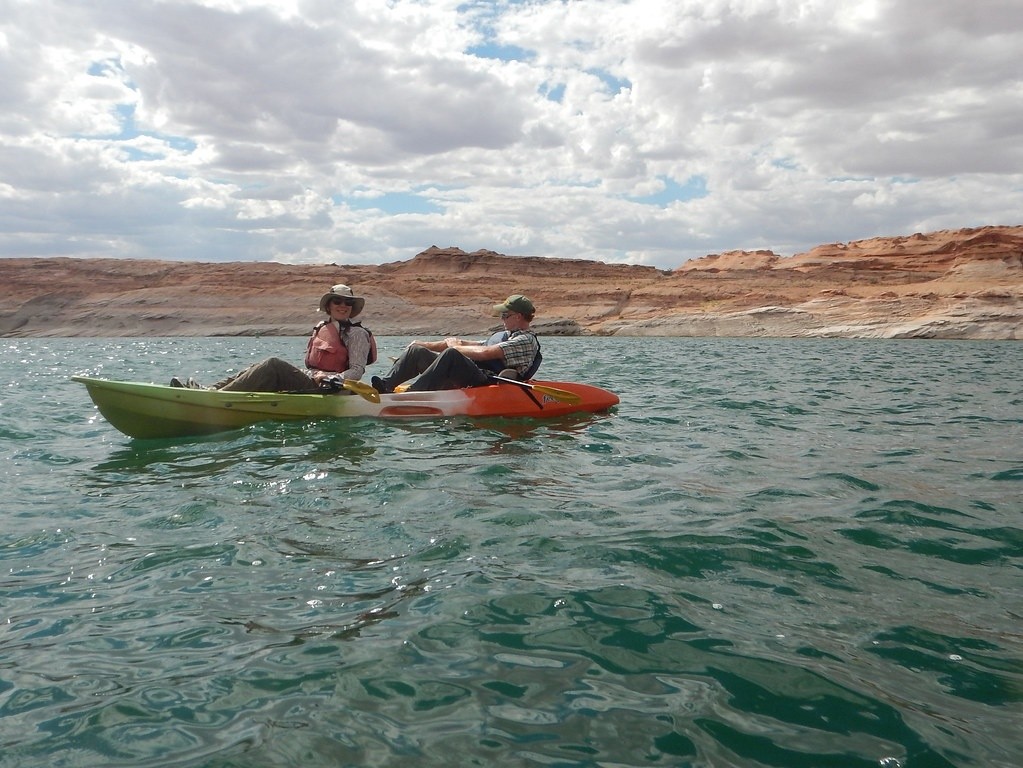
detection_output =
[371,375,387,394]
[186,377,213,390]
[169,376,186,387]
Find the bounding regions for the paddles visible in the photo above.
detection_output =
[388,355,582,403]
[310,375,381,404]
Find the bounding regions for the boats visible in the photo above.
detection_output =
[69,372,622,442]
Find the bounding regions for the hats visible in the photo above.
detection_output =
[493,295,534,319]
[316,284,365,319]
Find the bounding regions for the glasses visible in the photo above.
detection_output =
[500,312,513,318]
[328,296,356,307]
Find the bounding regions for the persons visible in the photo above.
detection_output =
[370,295,540,395]
[170,285,371,393]
